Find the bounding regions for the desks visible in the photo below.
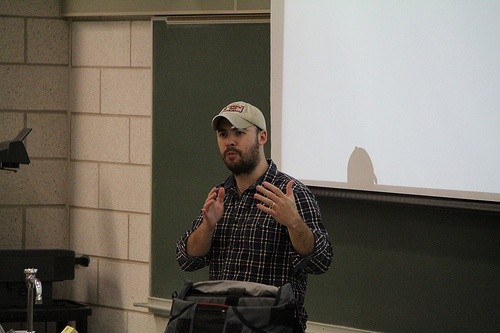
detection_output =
[0,299,92,333]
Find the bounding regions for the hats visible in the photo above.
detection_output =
[211,101,267,130]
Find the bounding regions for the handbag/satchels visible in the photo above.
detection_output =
[166,279,298,332]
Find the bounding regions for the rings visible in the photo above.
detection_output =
[270,202,275,208]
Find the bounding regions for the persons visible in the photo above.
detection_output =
[175,101,333,333]
